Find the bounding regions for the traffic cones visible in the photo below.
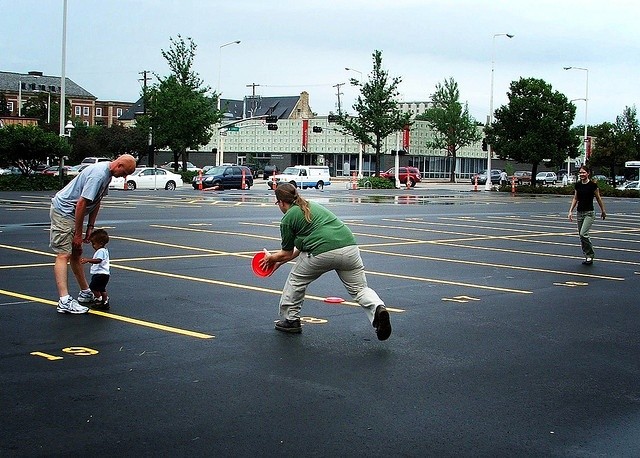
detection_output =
[474,174,477,192]
[407,169,410,189]
[241,166,246,190]
[511,175,516,193]
[124,175,128,190]
[352,170,359,190]
[273,170,276,191]
[198,169,203,190]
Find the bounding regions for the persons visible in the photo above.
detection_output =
[80,229,110,309]
[568,166,607,265]
[258,183,392,340]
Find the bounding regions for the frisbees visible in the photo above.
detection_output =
[324,296,344,304]
[251,252,274,278]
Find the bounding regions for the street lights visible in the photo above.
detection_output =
[563,66,590,167]
[567,98,588,174]
[59,120,76,189]
[344,67,363,180]
[485,33,514,191]
[215,40,242,168]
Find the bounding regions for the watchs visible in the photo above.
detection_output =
[48,154,136,314]
[87,223,94,227]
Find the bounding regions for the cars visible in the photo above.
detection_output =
[263,165,281,180]
[81,157,113,164]
[108,167,183,190]
[161,162,203,172]
[191,166,253,190]
[471,170,505,184]
[50,166,80,175]
[534,172,558,184]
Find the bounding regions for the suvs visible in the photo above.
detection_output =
[373,167,422,188]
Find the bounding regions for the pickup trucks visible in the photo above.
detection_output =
[507,171,535,184]
[269,166,331,190]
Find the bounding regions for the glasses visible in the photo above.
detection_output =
[275,200,283,205]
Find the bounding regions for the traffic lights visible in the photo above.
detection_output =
[265,115,279,123]
[398,150,406,157]
[313,126,323,133]
[390,149,398,156]
[267,124,279,131]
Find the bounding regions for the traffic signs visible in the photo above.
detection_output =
[229,126,240,132]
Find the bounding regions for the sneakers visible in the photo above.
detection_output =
[77,288,97,302]
[275,318,302,333]
[56,295,89,315]
[373,305,392,341]
